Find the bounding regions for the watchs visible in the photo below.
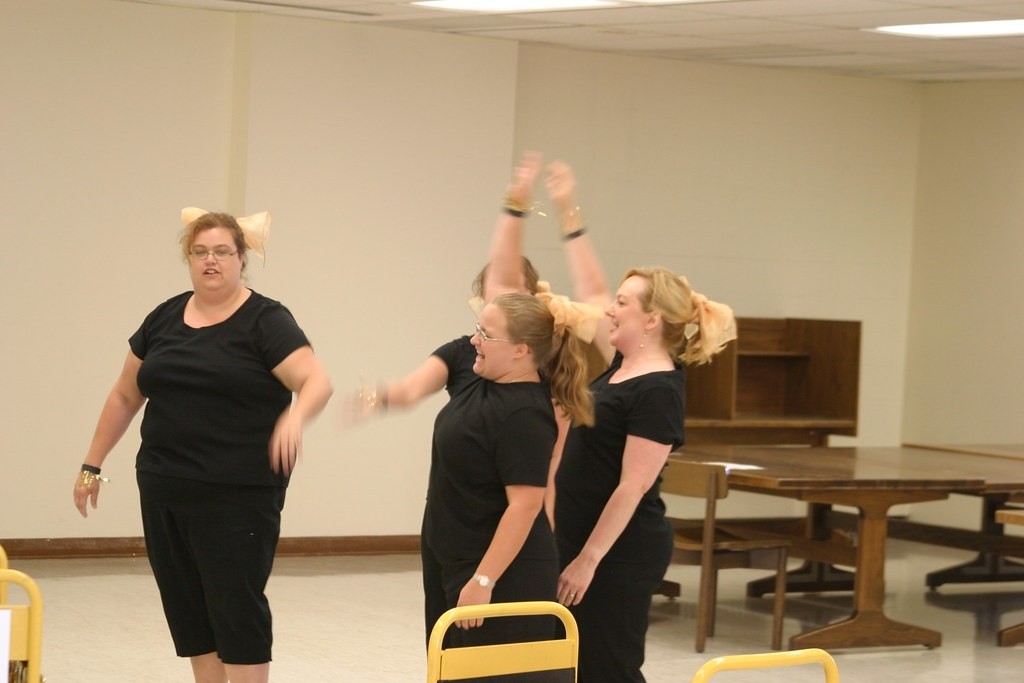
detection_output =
[474,572,496,590]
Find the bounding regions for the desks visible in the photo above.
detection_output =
[671,443,1024,652]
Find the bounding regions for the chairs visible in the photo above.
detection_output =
[656,462,788,654]
[691,646,839,683]
[427,600,580,683]
[0,543,43,682]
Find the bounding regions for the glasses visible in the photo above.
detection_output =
[188,248,238,261]
[476,322,516,342]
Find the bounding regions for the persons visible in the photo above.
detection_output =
[547,158,737,683]
[420,147,560,683]
[73,207,333,683]
[353,258,574,534]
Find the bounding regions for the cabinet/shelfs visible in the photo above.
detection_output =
[678,317,861,448]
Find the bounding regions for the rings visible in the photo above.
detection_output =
[568,593,575,598]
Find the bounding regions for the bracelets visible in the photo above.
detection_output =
[501,195,546,219]
[365,389,390,411]
[82,464,109,488]
[563,226,588,242]
